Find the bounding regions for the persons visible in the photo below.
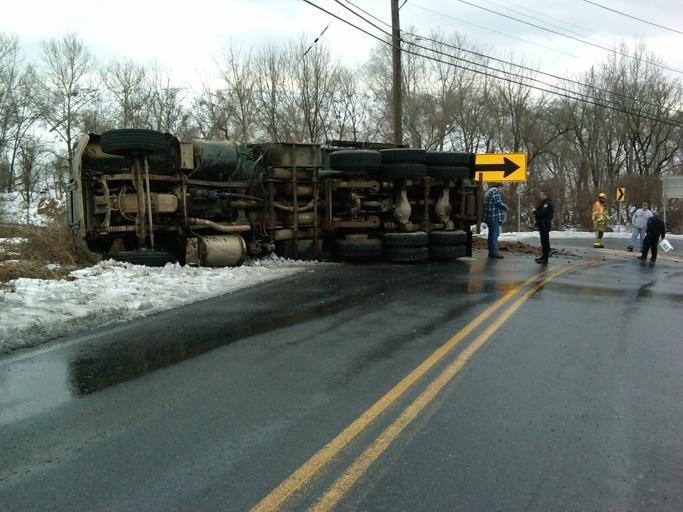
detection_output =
[590,191,610,249]
[530,190,554,264]
[627,200,652,253]
[636,209,665,263]
[481,182,509,261]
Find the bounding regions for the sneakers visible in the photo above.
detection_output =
[488,254,504,259]
[535,257,548,263]
[594,243,605,249]
[637,256,647,260]
[650,258,656,262]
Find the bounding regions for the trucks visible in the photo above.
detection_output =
[64,125,486,266]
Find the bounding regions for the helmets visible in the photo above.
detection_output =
[599,193,607,199]
